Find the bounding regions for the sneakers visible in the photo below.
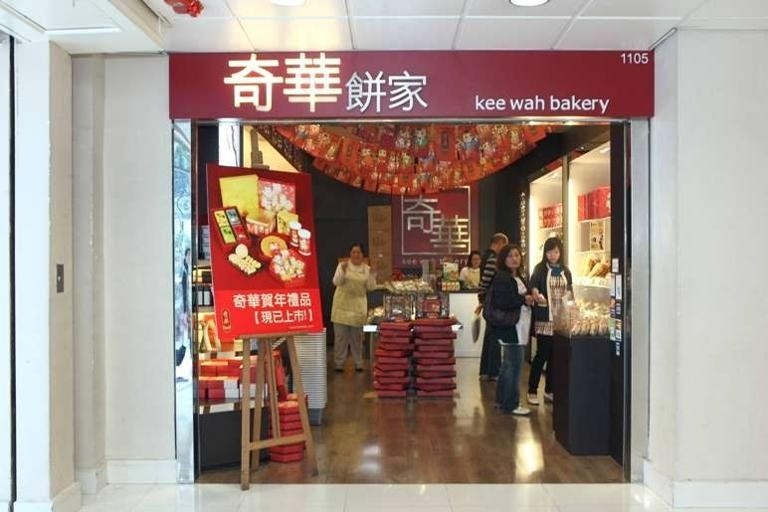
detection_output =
[526,392,539,405]
[512,406,531,415]
[543,393,553,402]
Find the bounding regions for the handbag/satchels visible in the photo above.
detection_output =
[482,291,519,328]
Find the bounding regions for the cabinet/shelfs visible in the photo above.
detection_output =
[526,139,610,327]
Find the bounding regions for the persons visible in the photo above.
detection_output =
[477,233,509,381]
[527,237,573,405]
[482,244,535,415]
[459,250,483,290]
[330,242,376,373]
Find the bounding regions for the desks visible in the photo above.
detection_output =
[554,330,610,454]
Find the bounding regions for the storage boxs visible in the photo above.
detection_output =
[338,206,393,278]
